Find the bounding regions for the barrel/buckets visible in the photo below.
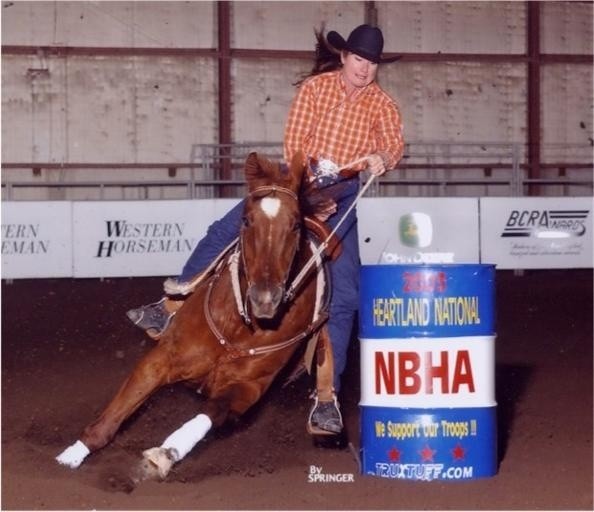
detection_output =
[357,263,498,482]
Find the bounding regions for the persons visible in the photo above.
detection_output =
[127,25,404,433]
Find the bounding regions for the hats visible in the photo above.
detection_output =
[327,23,402,64]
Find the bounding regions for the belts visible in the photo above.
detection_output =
[309,156,360,178]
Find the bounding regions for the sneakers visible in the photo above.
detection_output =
[307,400,342,434]
[126,300,170,332]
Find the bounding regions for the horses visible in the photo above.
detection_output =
[54,150,326,480]
